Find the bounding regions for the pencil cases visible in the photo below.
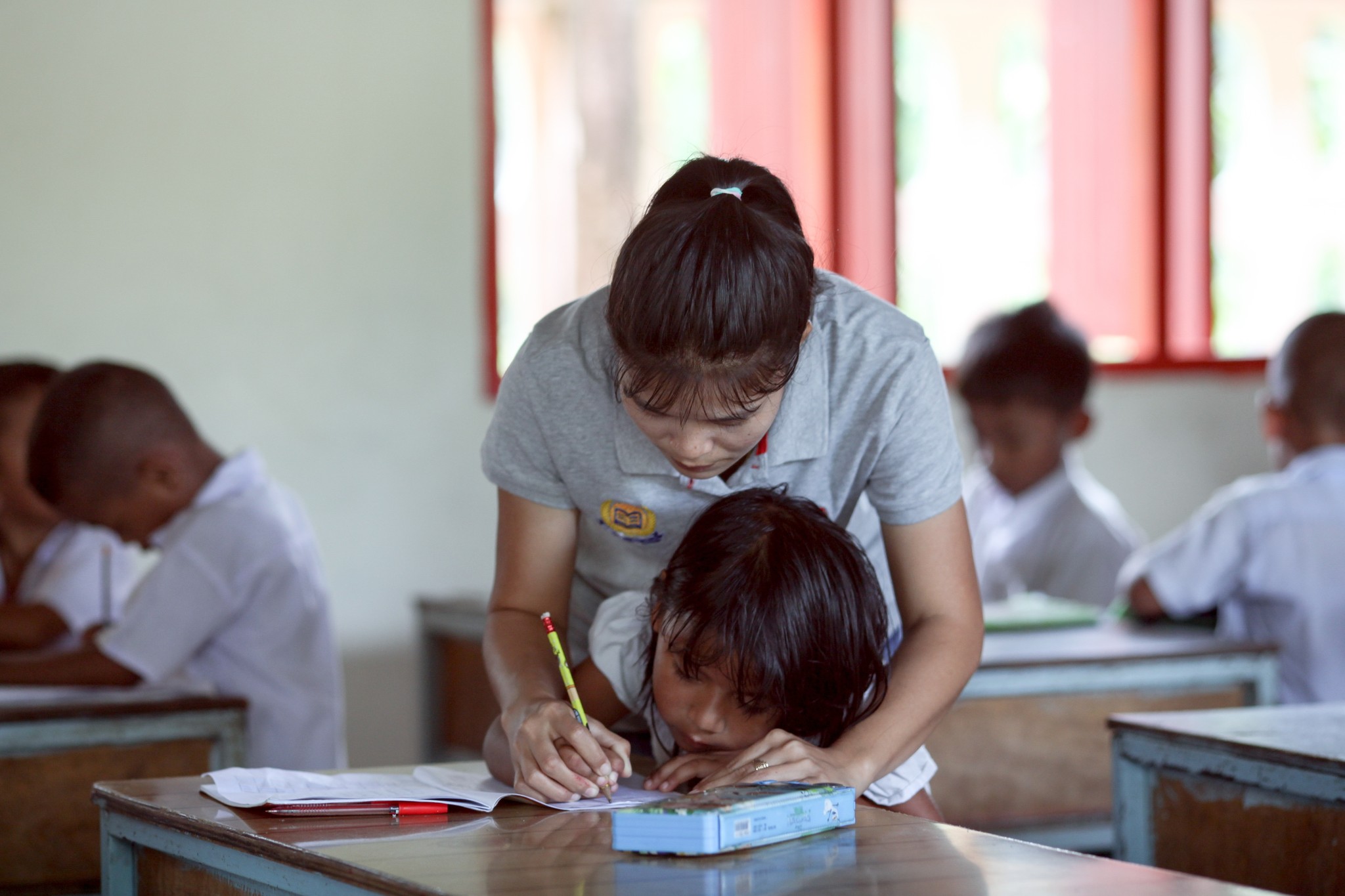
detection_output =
[608,775,860,856]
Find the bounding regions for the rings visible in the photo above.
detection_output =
[754,759,769,771]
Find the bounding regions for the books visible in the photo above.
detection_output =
[981,593,1102,631]
[197,764,684,813]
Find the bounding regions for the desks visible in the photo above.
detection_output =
[415,592,1281,863]
[1106,697,1345,896]
[0,678,256,896]
[90,753,1284,896]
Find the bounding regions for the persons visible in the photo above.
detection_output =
[956,302,1136,665]
[1113,313,1345,705]
[0,358,346,775]
[479,154,985,806]
[482,489,948,824]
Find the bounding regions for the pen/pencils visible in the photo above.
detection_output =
[265,801,450,816]
[541,611,614,804]
